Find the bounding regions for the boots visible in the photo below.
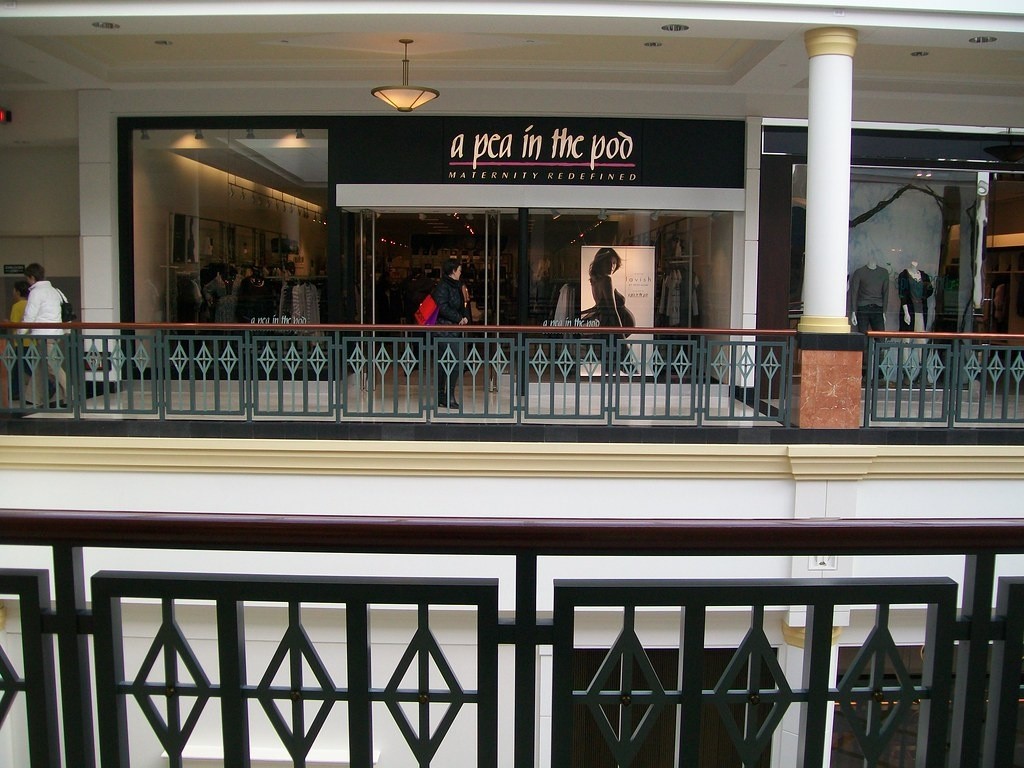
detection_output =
[444,371,459,409]
[438,371,447,408]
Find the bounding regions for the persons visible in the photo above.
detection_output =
[590,248,635,375]
[850,254,895,388]
[430,258,471,410]
[4,263,76,405]
[898,261,933,389]
[475,268,496,309]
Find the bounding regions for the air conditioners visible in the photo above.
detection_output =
[296,128,306,140]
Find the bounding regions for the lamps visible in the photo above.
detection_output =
[141,128,150,141]
[194,128,203,141]
[371,39,440,112]
[598,209,608,221]
[227,182,327,225]
[650,210,660,221]
[466,214,473,220]
[246,128,255,140]
[550,208,561,220]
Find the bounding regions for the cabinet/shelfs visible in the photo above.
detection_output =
[984,245,1024,344]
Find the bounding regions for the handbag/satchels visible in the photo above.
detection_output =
[414,294,440,325]
[57,289,77,322]
